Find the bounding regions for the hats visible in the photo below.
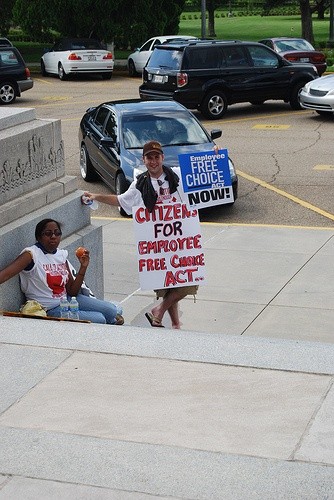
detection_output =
[143,141,163,156]
[20,300,47,317]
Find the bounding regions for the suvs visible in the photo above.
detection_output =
[0,45,34,105]
[140,39,318,120]
[0,38,13,48]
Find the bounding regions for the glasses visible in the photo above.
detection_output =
[41,231,62,237]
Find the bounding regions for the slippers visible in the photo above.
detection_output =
[145,312,165,328]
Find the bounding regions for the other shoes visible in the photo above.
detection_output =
[115,315,124,325]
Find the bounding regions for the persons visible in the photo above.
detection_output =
[0,218,124,325]
[83,141,218,330]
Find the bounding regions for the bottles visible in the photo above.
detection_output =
[82,195,98,210]
[69,297,79,320]
[60,297,71,319]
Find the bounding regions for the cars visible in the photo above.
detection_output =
[78,97,240,218]
[253,37,327,77]
[299,74,334,115]
[127,35,202,77]
[40,36,114,81]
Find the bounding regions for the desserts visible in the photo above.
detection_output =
[75,247,86,258]
